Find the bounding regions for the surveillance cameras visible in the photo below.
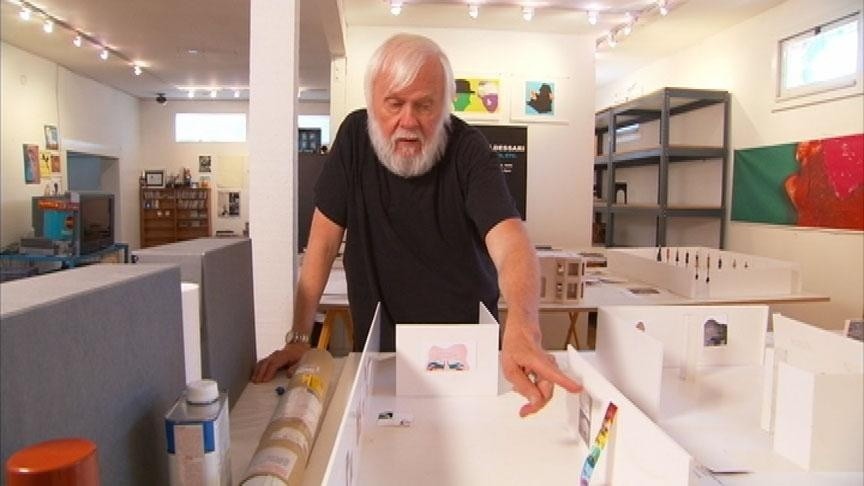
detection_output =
[156,97,167,103]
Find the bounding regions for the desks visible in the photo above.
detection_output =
[312,247,832,350]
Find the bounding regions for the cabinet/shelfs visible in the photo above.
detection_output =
[592,87,729,261]
[139,182,211,249]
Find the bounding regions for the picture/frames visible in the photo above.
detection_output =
[21,123,63,183]
[144,170,165,188]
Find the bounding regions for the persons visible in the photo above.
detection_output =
[244,30,585,420]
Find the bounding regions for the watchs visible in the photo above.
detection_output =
[283,330,310,346]
